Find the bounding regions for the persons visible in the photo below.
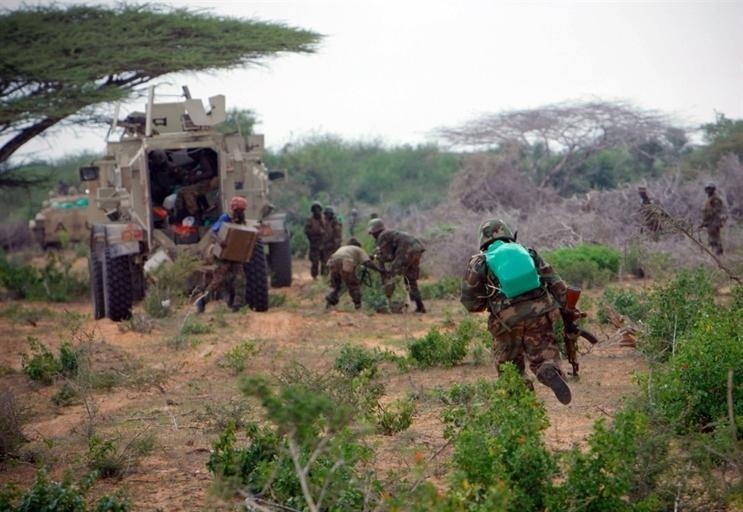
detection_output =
[305,202,428,314]
[699,181,728,256]
[173,149,220,229]
[460,219,579,407]
[192,196,258,315]
[635,186,663,234]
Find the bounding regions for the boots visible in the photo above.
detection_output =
[195,296,205,313]
[415,305,425,313]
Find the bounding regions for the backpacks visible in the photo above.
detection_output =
[483,239,541,299]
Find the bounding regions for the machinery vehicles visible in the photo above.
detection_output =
[79,84,293,323]
[27,186,87,251]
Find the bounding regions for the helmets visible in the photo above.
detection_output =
[367,218,385,233]
[705,183,715,191]
[231,197,248,210]
[323,207,334,214]
[479,219,513,249]
[312,204,321,211]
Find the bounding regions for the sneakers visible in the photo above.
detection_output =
[541,365,571,405]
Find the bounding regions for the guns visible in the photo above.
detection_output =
[558,285,598,376]
